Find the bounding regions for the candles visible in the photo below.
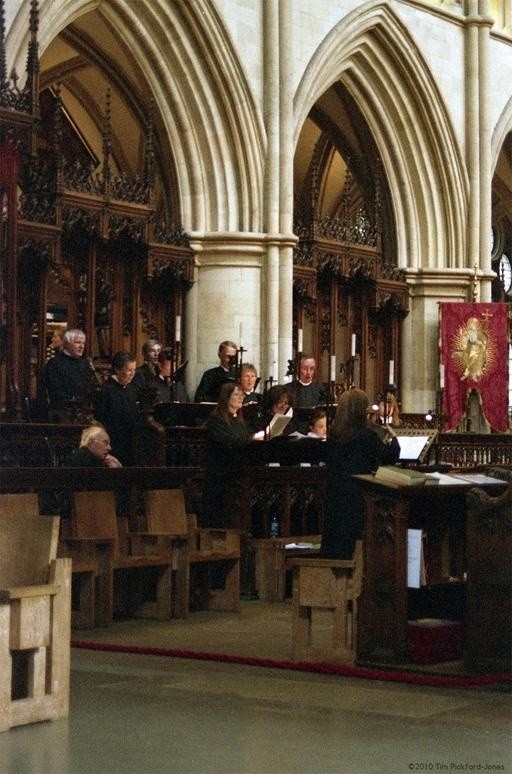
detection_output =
[351,334,356,357]
[388,360,394,383]
[330,355,336,381]
[175,316,181,340]
[439,363,445,389]
[297,329,303,352]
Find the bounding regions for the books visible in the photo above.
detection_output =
[425,474,438,484]
[374,464,428,485]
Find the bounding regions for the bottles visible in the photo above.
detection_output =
[269,517,279,539]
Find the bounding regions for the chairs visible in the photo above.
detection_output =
[0,489,365,734]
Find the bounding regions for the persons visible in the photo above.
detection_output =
[46,327,189,514]
[193,341,399,558]
